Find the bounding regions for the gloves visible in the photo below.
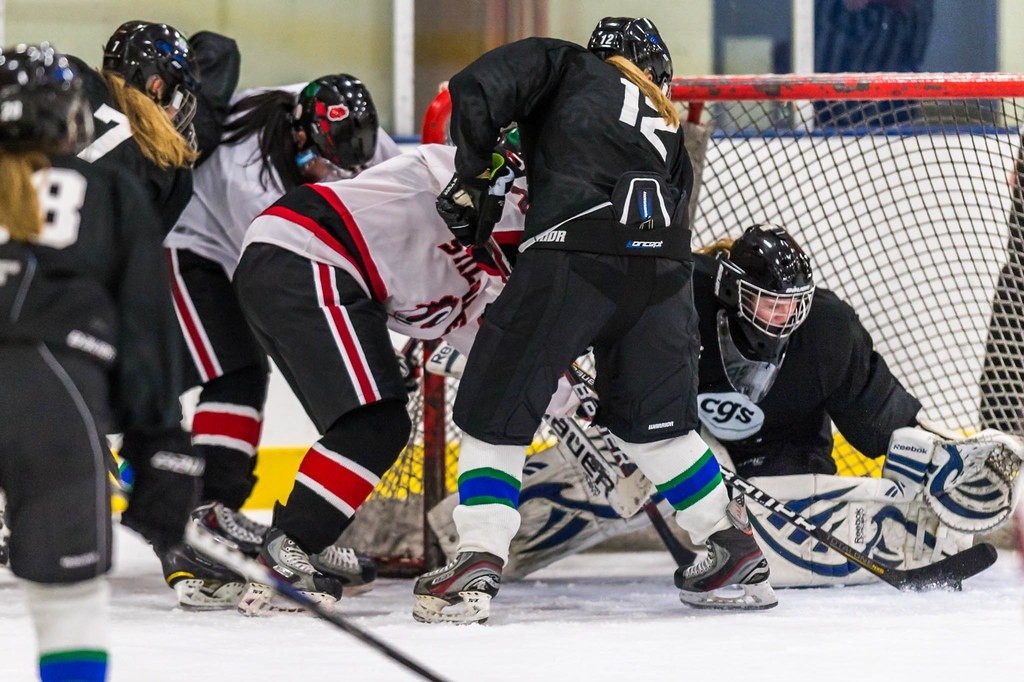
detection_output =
[436,151,515,250]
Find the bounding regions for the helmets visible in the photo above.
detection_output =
[714,222,816,344]
[0,44,74,153]
[293,74,378,171]
[103,20,197,134]
[494,124,527,179]
[588,17,673,100]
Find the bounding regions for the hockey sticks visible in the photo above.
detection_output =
[182,521,453,681]
[451,187,999,596]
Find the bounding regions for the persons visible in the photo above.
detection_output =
[0,17,1024,682]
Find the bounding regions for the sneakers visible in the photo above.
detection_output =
[191,500,270,560]
[674,492,778,610]
[161,540,247,611]
[412,551,505,625]
[238,498,342,617]
[309,545,376,597]
